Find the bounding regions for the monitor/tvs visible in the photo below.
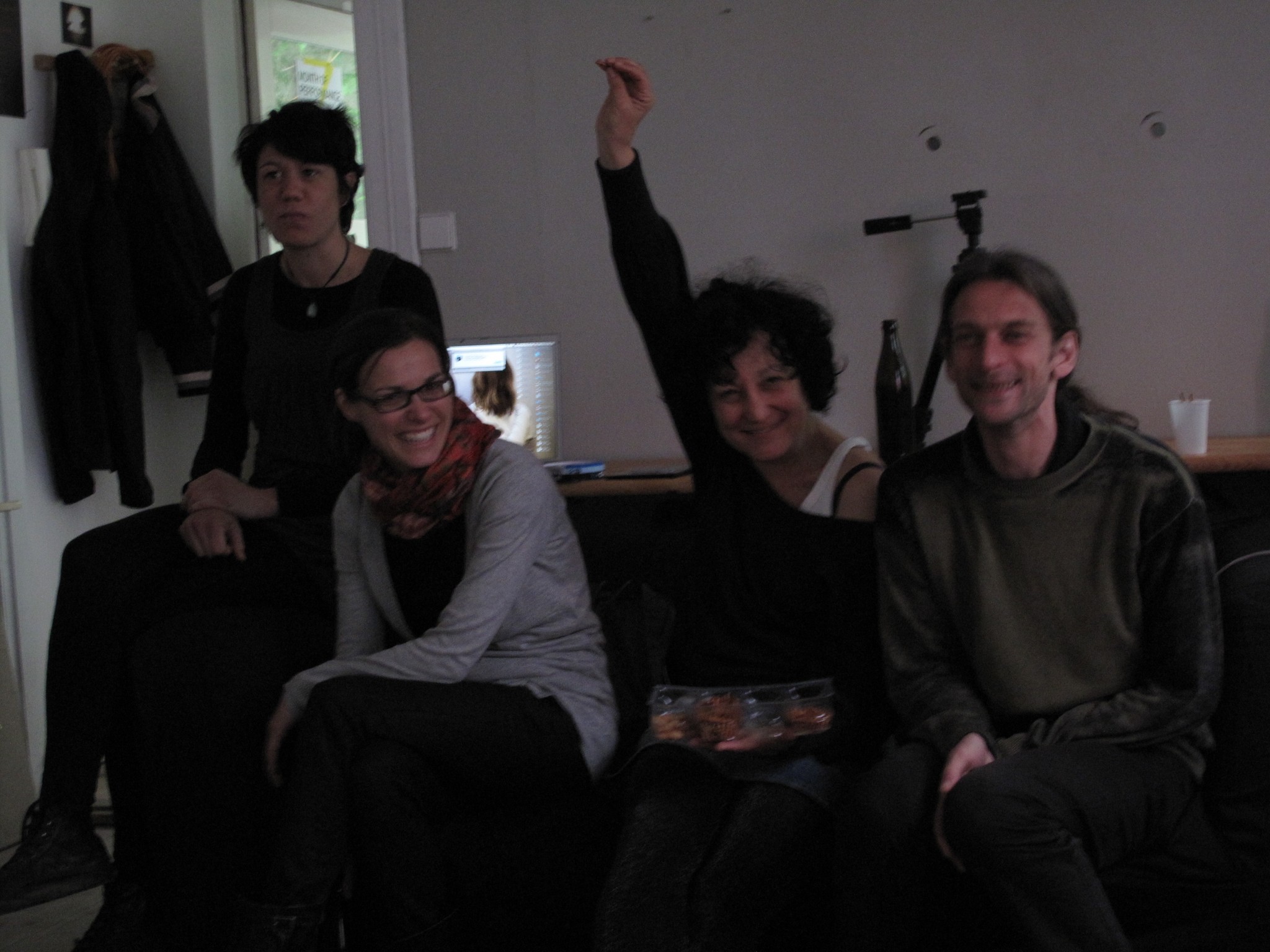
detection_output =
[442,333,563,466]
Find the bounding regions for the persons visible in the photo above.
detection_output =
[267,307,620,952]
[0,102,447,952]
[875,249,1224,952]
[592,54,888,952]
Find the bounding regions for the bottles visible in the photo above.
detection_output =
[875,320,915,466]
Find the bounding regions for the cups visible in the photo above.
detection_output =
[1168,398,1212,457]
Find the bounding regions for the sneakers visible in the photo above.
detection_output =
[0,801,111,914]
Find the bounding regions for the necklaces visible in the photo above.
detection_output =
[283,236,348,319]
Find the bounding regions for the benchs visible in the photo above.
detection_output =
[75,436,1270,952]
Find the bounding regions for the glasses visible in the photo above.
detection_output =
[354,371,454,415]
